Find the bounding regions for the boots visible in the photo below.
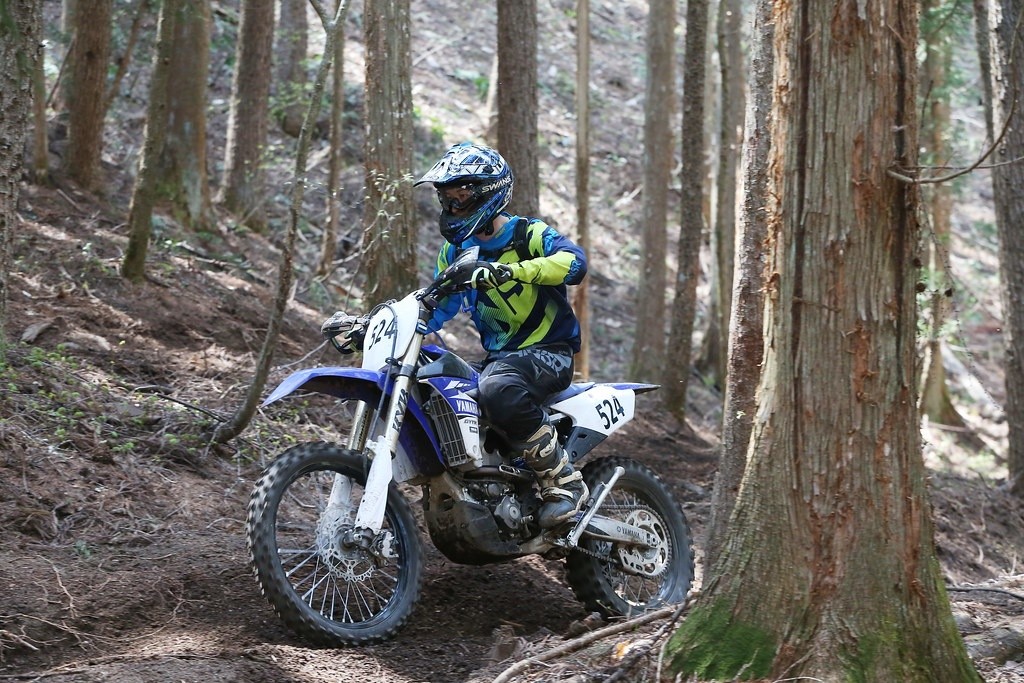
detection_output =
[517,408,589,528]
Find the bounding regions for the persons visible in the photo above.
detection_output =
[330,140,590,528]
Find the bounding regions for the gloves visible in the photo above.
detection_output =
[344,330,365,353]
[454,261,513,293]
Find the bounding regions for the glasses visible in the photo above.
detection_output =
[437,172,512,211]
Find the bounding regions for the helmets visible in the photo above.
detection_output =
[413,143,514,245]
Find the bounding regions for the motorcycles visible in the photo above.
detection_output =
[246,247,696,648]
